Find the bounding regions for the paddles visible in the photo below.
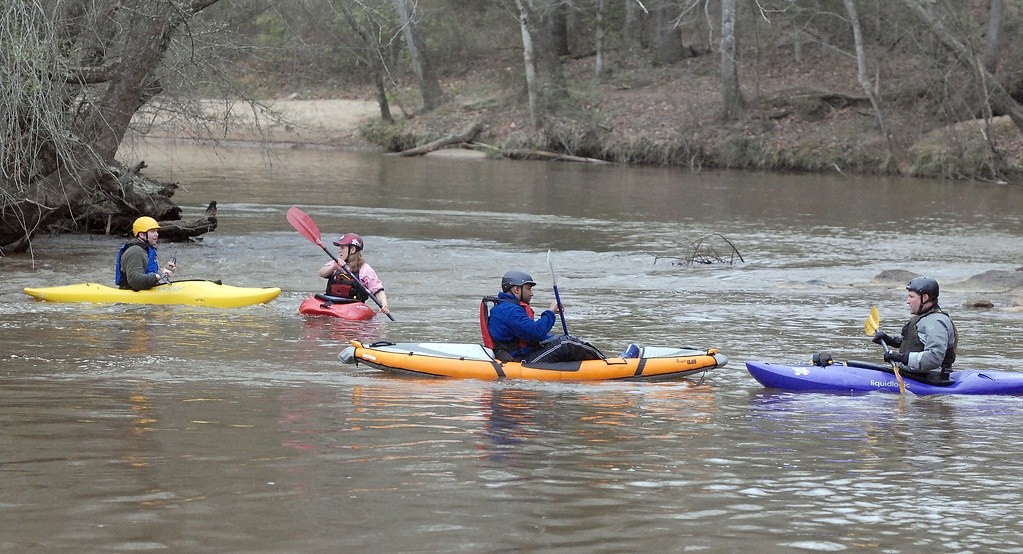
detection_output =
[286,207,396,321]
[158,251,177,286]
[544,249,570,334]
[864,306,905,396]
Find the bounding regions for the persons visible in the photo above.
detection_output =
[119,216,176,290]
[319,233,390,317]
[872,277,959,384]
[488,269,641,365]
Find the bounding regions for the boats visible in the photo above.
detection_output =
[297,293,378,322]
[335,338,730,384]
[22,278,282,310]
[746,356,1023,401]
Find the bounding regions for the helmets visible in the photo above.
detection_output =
[133,217,161,237]
[502,270,536,293]
[906,276,939,298]
[333,232,363,250]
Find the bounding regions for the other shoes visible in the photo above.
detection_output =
[618,342,644,359]
[814,350,834,367]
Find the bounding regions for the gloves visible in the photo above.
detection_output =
[884,351,910,366]
[872,332,901,348]
